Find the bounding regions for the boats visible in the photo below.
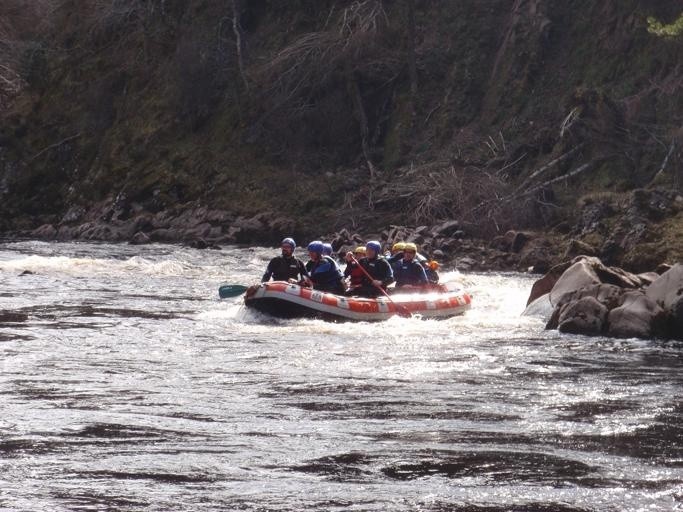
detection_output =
[244,281,472,323]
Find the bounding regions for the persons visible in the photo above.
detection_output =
[261,238,440,300]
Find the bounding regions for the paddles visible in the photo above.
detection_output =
[350,254,413,318]
[219,285,249,298]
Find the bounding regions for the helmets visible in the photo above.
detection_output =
[280,237,297,253]
[308,241,324,255]
[354,246,367,255]
[392,242,418,252]
[324,243,333,256]
[367,240,381,254]
